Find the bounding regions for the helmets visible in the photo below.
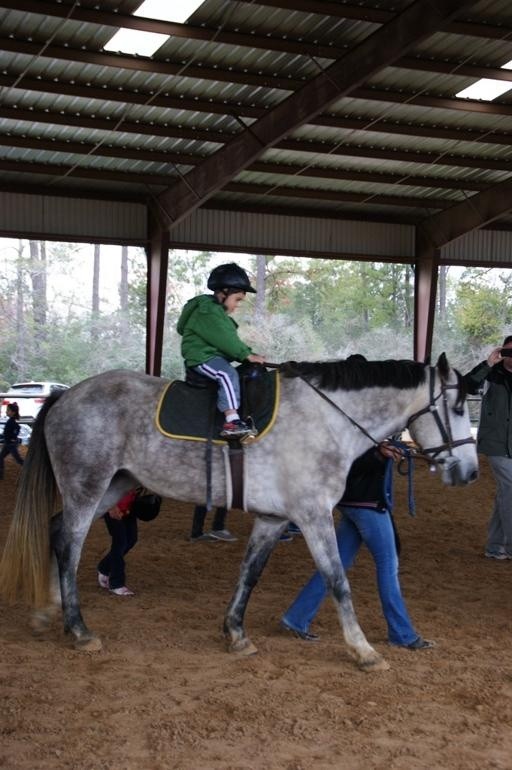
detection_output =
[206,262,257,294]
[127,493,162,522]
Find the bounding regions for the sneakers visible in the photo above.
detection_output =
[403,637,436,649]
[208,528,241,543]
[190,532,211,542]
[279,620,321,642]
[110,585,135,597]
[219,421,258,436]
[287,522,302,534]
[483,549,512,560]
[97,568,110,589]
[276,534,293,542]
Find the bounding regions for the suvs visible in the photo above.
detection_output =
[2,382,72,426]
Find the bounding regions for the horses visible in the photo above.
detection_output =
[0,352,481,673]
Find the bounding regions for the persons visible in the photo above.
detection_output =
[279,354,436,650]
[97,478,151,596]
[460,336,512,559]
[177,263,264,437]
[190,503,239,544]
[0,404,24,479]
[277,522,302,541]
